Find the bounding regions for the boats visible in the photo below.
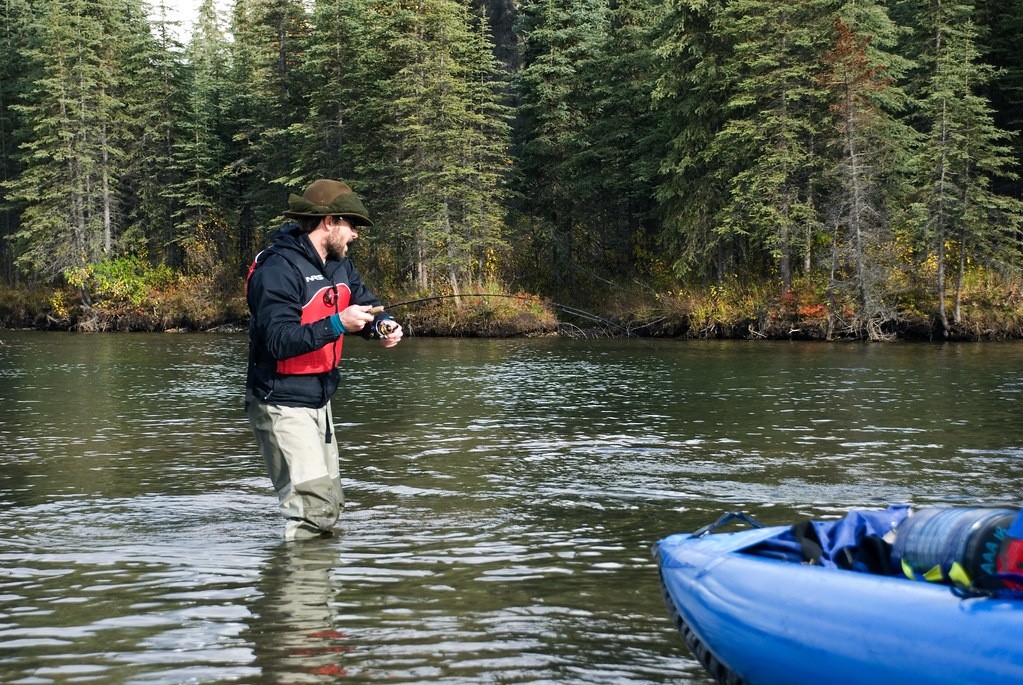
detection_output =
[653,505,1023,685]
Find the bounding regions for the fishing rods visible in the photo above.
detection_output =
[368,292,626,330]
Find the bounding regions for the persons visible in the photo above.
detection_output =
[244,180,403,488]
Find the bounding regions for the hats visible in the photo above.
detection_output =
[282,180,375,227]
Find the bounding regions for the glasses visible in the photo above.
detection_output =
[339,215,358,229]
